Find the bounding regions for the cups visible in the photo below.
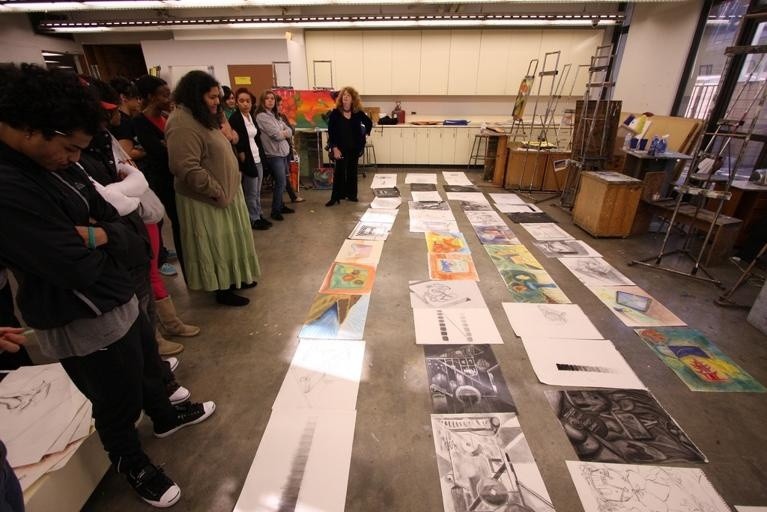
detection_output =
[628,135,637,150]
[638,136,646,149]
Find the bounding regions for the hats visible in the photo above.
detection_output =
[75,71,120,113]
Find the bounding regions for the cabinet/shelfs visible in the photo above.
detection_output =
[484,113,765,272]
[320,123,576,165]
[299,26,608,102]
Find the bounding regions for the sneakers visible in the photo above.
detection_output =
[158,262,177,277]
[229,280,257,291]
[215,291,250,307]
[116,450,183,510]
[293,196,305,202]
[279,206,295,214]
[166,250,177,258]
[270,208,284,221]
[249,214,272,231]
[161,356,179,372]
[167,385,192,406]
[147,398,219,441]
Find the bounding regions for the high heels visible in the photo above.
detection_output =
[325,197,340,206]
[344,195,360,203]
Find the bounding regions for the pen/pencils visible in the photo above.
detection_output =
[0,328,35,354]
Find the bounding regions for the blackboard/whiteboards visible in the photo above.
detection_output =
[274,62,292,89]
[168,66,212,94]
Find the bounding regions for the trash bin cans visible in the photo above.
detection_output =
[289,159,300,193]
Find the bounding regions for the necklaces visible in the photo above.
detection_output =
[343,108,351,113]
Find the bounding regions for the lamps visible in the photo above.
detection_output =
[25,2,638,39]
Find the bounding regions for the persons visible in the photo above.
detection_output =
[69,70,200,404]
[0,441,25,512]
[166,71,260,306]
[1,63,217,507]
[219,90,305,230]
[325,86,373,206]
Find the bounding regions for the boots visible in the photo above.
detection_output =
[146,326,185,357]
[151,294,202,339]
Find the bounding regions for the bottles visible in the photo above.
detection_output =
[647,135,665,156]
[391,101,405,124]
[750,168,766,185]
